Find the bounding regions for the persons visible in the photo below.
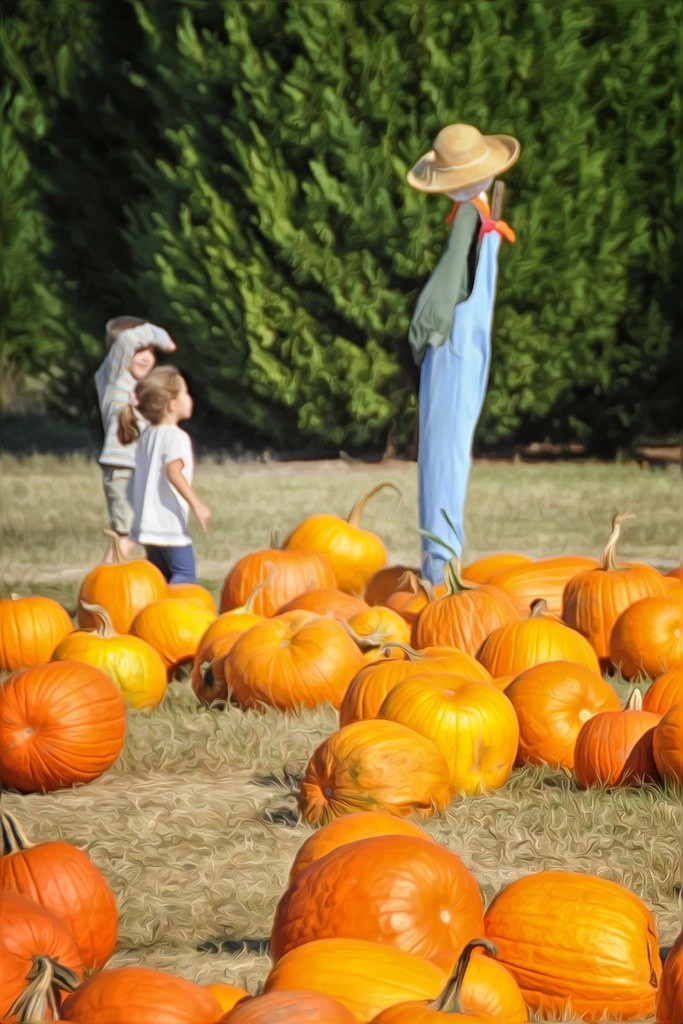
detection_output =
[116,364,210,584]
[407,124,520,578]
[94,315,177,563]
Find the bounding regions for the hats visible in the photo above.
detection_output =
[404,122,522,194]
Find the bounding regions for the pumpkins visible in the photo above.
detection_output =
[0,483,683,1024]
